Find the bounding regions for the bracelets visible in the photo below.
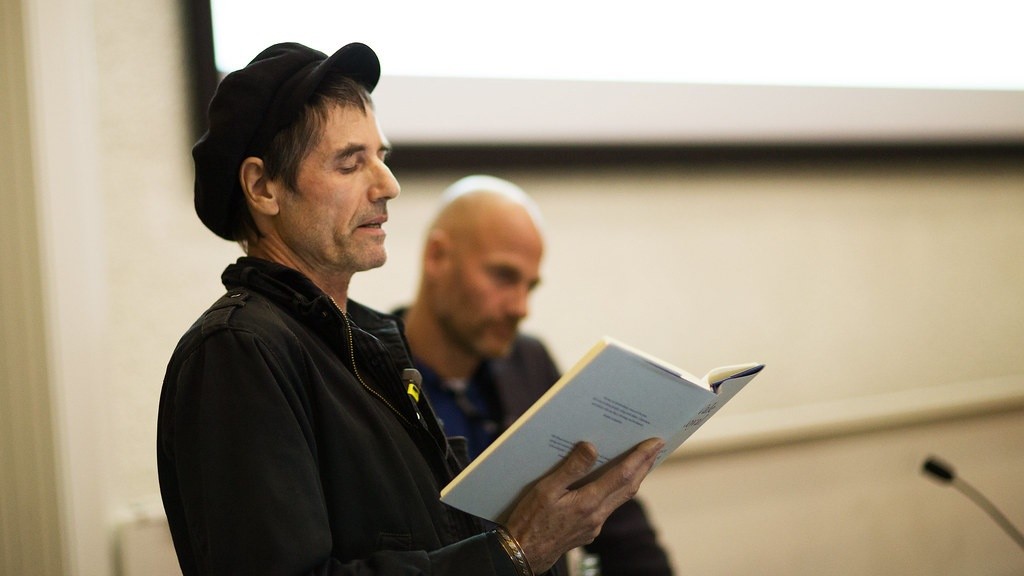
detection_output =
[492,524,534,576]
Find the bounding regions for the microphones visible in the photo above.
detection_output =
[921,456,1024,550]
[403,368,422,387]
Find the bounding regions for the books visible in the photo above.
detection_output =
[439,334,766,527]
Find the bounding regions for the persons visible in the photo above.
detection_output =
[392,176,676,576]
[156,38,666,574]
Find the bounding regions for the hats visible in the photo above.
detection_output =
[192,42,381,241]
